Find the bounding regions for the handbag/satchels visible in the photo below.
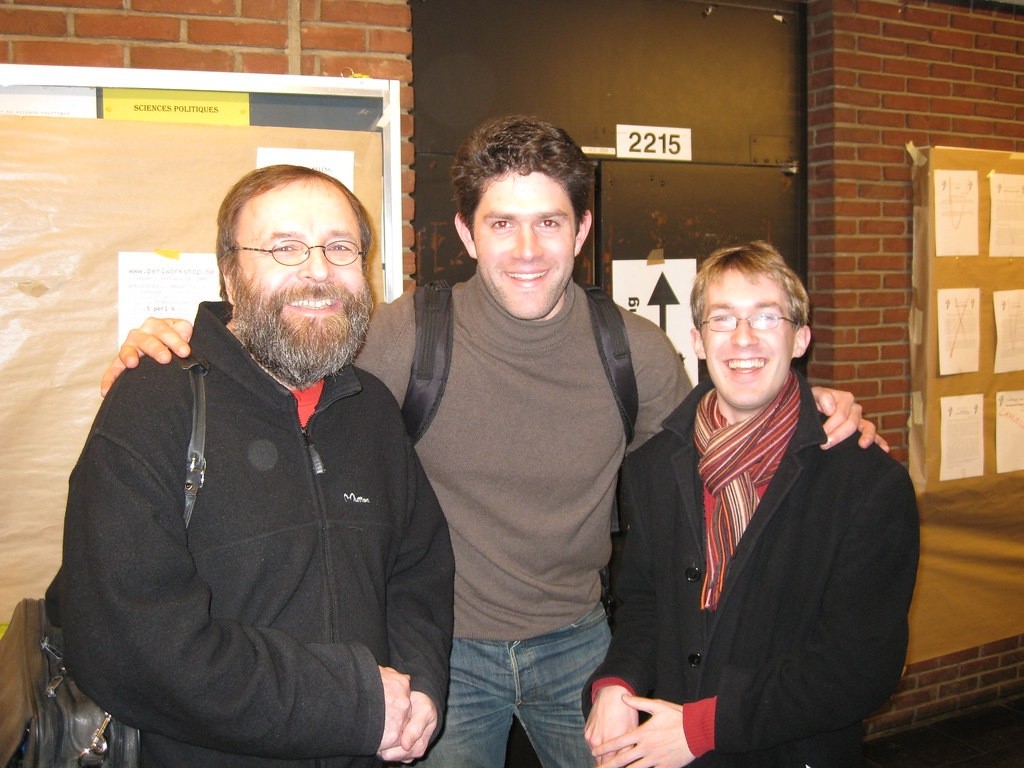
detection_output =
[0,355,209,768]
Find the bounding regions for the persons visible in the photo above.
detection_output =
[100,116,890,768]
[582,240,919,768]
[42,165,455,768]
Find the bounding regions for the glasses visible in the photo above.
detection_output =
[698,311,799,333]
[226,239,367,266]
